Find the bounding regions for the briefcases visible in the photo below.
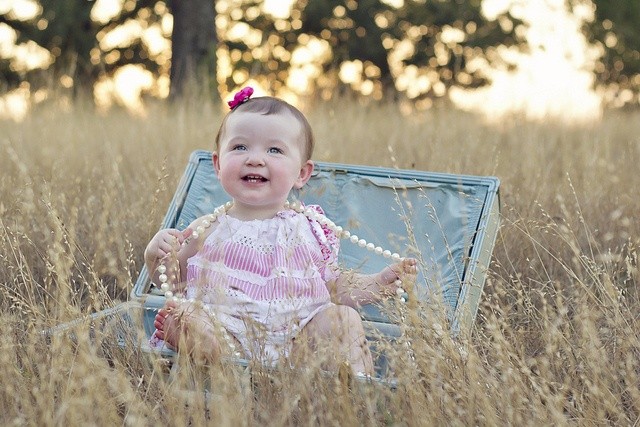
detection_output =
[37,149,502,403]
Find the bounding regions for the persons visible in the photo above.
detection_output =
[143,95,419,379]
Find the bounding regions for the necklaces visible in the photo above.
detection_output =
[157,198,420,374]
